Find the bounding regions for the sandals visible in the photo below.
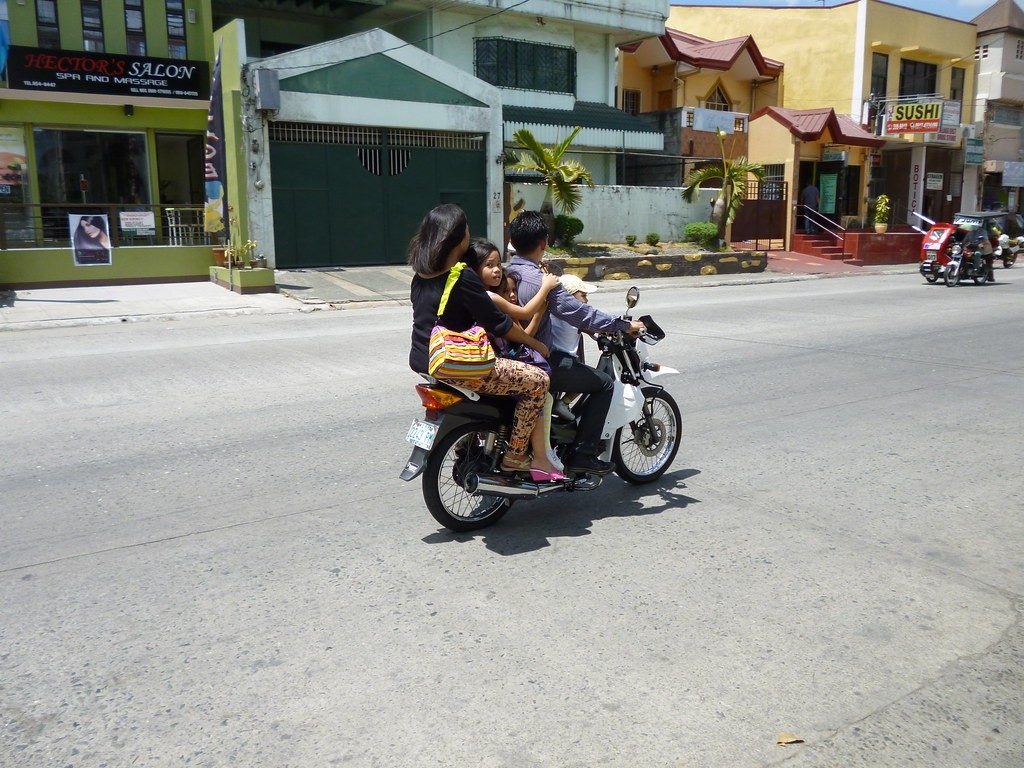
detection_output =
[500,455,532,471]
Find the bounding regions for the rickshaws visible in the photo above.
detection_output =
[916,224,994,288]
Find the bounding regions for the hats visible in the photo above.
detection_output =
[557,274,598,295]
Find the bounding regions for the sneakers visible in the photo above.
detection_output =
[551,399,576,420]
[545,445,564,471]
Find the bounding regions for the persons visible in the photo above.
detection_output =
[962,229,989,274]
[801,185,819,234]
[408,202,647,492]
[74,216,108,264]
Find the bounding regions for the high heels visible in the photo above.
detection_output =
[529,466,570,481]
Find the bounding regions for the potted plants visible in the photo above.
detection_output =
[224,240,258,269]
[874,195,891,233]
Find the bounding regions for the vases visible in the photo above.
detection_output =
[212,248,234,268]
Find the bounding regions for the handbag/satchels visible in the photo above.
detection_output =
[429,261,496,379]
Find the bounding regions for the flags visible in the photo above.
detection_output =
[203,47,229,233]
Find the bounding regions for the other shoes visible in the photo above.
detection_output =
[570,452,617,474]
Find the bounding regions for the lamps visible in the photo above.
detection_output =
[124,105,134,117]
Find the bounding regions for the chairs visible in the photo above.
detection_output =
[164,207,212,246]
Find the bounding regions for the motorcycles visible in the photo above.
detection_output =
[398,286,683,535]
[953,211,1024,270]
